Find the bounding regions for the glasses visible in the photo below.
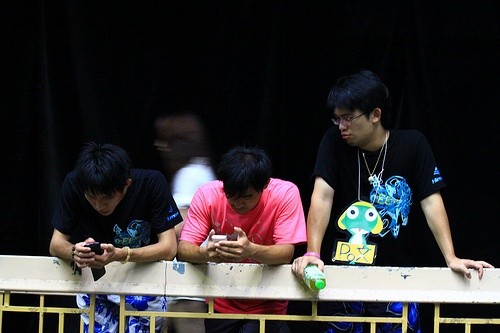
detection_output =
[331,112,365,125]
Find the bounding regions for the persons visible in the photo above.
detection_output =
[177,146,306,333]
[48,142,183,333]
[291,70,495,333]
[157,113,219,333]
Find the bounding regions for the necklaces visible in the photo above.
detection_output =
[362,130,389,182]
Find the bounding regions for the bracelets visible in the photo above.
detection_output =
[120,245,131,264]
[72,244,75,263]
[303,252,321,259]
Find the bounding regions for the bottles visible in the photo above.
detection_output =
[301,263,326,291]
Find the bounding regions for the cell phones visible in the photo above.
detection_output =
[83,242,103,256]
[211,234,228,243]
